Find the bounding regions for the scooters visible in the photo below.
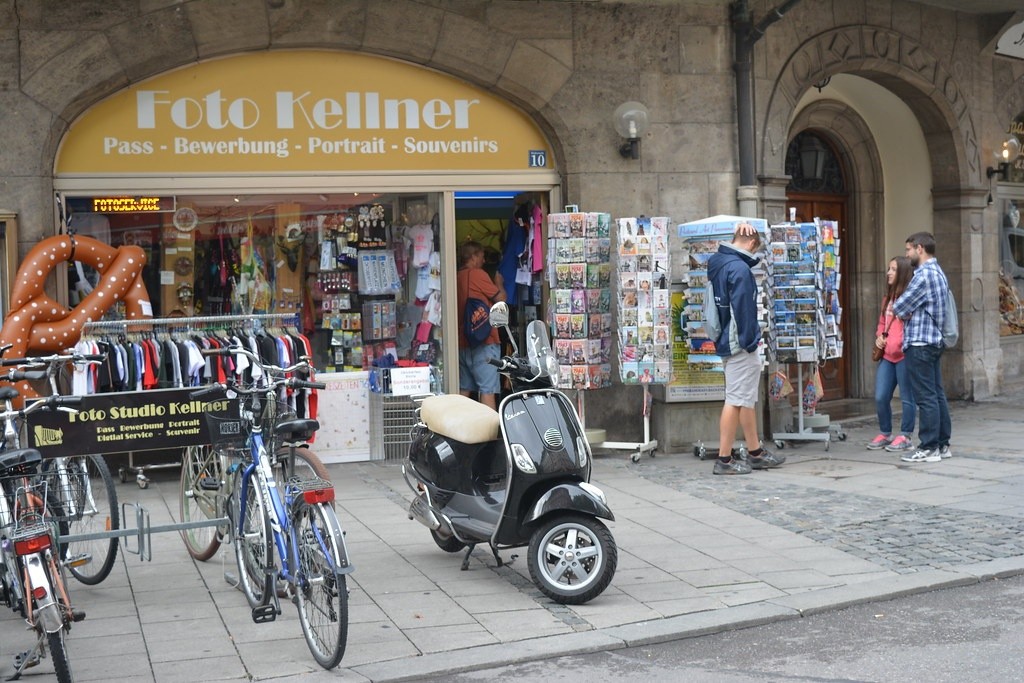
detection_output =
[403,301,618,604]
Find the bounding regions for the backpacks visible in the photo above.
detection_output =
[464,268,492,350]
[702,280,731,342]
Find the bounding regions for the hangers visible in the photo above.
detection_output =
[81,313,299,342]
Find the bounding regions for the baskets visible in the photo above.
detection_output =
[0,463,89,529]
[203,398,297,458]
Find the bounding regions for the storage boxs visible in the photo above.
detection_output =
[363,302,396,342]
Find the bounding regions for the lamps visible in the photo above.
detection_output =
[986,133,1020,182]
[796,135,828,182]
[813,76,831,93]
[613,101,652,160]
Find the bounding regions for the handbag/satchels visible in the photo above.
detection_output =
[918,268,959,348]
[872,343,886,362]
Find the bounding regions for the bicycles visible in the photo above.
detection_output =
[0,344,120,683]
[176,348,353,671]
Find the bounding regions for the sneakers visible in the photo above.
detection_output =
[901,446,942,463]
[746,440,786,469]
[866,433,893,449]
[885,435,914,452]
[713,457,752,475]
[940,446,952,458]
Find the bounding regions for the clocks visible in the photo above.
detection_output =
[173,207,198,232]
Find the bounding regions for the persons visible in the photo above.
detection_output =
[705,223,787,474]
[621,221,670,383]
[456,241,508,413]
[867,256,917,450]
[890,231,953,461]
